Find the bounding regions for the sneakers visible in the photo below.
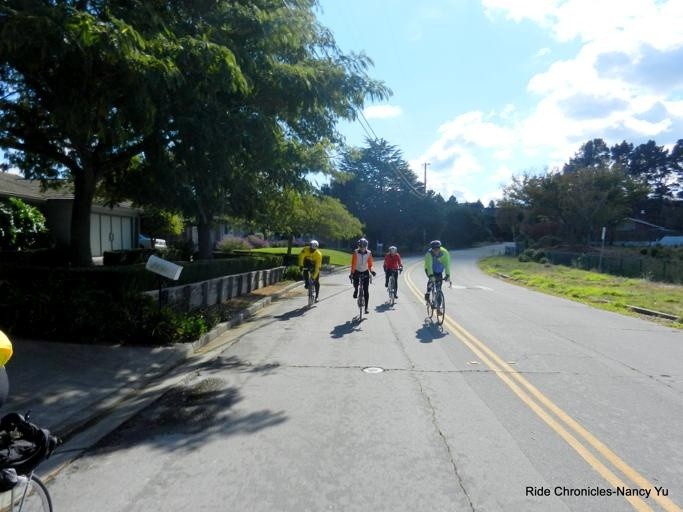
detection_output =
[315,297,319,302]
[425,294,429,300]
[366,310,368,313]
[437,310,442,315]
[394,295,398,298]
[385,284,388,287]
[354,292,357,297]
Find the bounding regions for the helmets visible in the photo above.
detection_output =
[389,246,397,253]
[358,239,368,247]
[310,240,319,250]
[431,241,441,249]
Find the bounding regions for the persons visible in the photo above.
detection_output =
[383,245,403,299]
[298,239,323,303]
[349,237,373,314]
[424,239,451,316]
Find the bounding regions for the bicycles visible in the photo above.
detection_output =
[423,275,447,327]
[0,414,61,510]
[348,273,374,322]
[298,265,320,305]
[384,266,402,306]
